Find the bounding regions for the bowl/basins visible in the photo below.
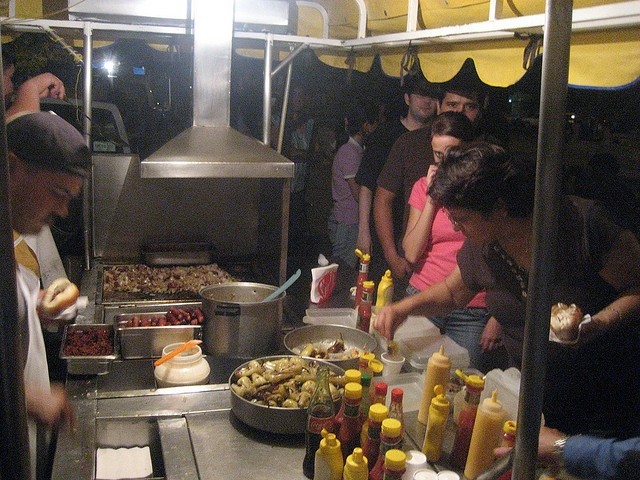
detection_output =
[229,355,346,435]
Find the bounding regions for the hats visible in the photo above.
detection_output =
[4,109,91,176]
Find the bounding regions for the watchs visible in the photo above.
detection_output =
[540,434,572,479]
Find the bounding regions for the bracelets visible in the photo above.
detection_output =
[601,306,625,334]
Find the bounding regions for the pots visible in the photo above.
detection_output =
[199,282,286,358]
[283,324,377,370]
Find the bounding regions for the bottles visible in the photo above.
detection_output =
[303,366,335,480]
[422,385,450,462]
[343,447,368,480]
[154,343,211,388]
[358,351,375,372]
[418,344,452,425]
[368,419,402,480]
[356,281,375,334]
[388,388,404,437]
[355,249,370,307]
[314,428,343,480]
[498,420,517,479]
[337,382,361,464]
[367,359,383,377]
[363,402,388,470]
[382,449,406,480]
[361,373,372,425]
[368,270,394,337]
[375,381,388,405]
[448,369,485,470]
[335,369,362,437]
[464,389,507,479]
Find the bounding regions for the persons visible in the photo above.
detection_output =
[1,52,66,123]
[354,72,443,281]
[231,69,337,286]
[401,110,505,373]
[426,139,640,434]
[5,110,93,480]
[326,101,380,292]
[371,75,504,302]
[492,424,640,480]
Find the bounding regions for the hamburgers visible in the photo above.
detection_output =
[39,277,80,320]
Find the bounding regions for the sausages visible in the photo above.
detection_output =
[165,312,179,326]
[132,315,139,327]
[178,309,191,321]
[150,315,157,327]
[185,306,196,318]
[169,306,183,320]
[194,307,204,323]
[159,314,165,327]
[141,315,148,327]
